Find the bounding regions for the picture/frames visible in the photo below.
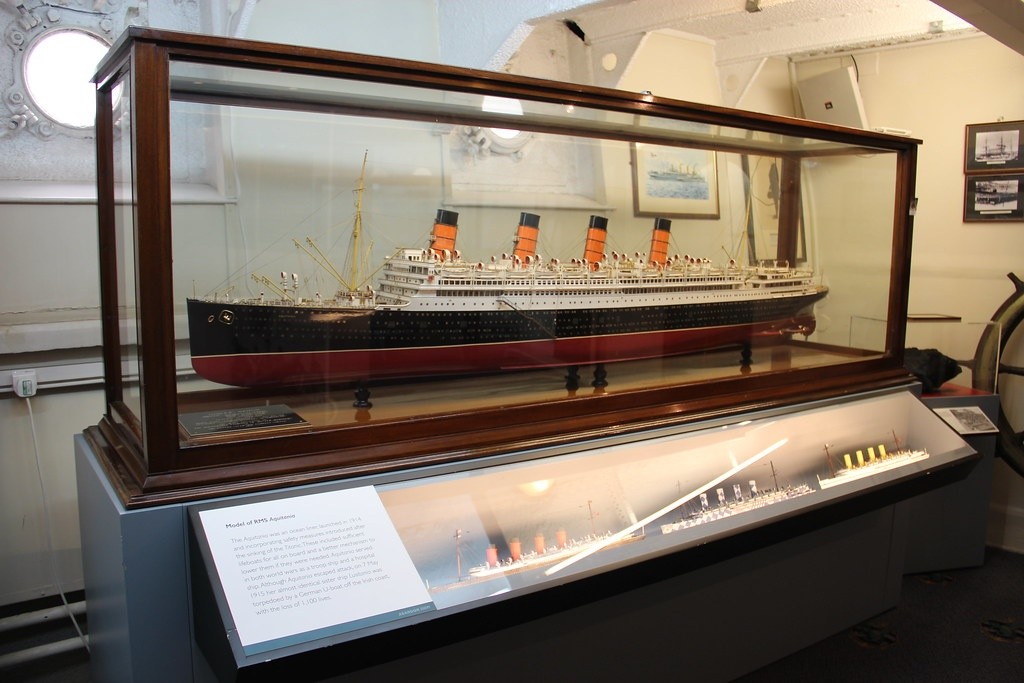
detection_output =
[633,113,720,137]
[964,120,1024,174]
[741,154,807,267]
[630,142,721,220]
[744,129,787,143]
[963,173,1024,222]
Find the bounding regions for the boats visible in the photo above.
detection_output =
[649,163,705,182]
[976,135,1017,162]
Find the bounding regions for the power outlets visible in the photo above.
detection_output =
[12,369,38,397]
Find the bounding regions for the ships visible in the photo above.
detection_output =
[186,150,829,389]
[426,500,646,595]
[816,429,929,490]
[660,460,816,534]
